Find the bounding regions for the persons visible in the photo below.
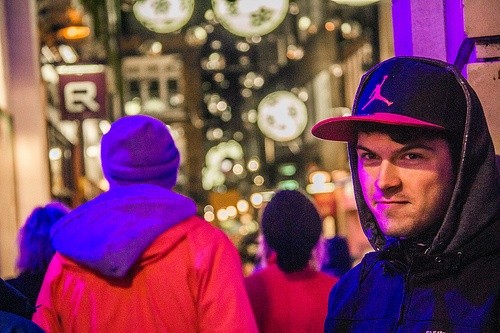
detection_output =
[32,115,259,333]
[1,202,78,333]
[312,54,500,333]
[241,189,357,333]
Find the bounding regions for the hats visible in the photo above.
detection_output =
[310,55,468,144]
[100,115,181,193]
[259,187,323,266]
